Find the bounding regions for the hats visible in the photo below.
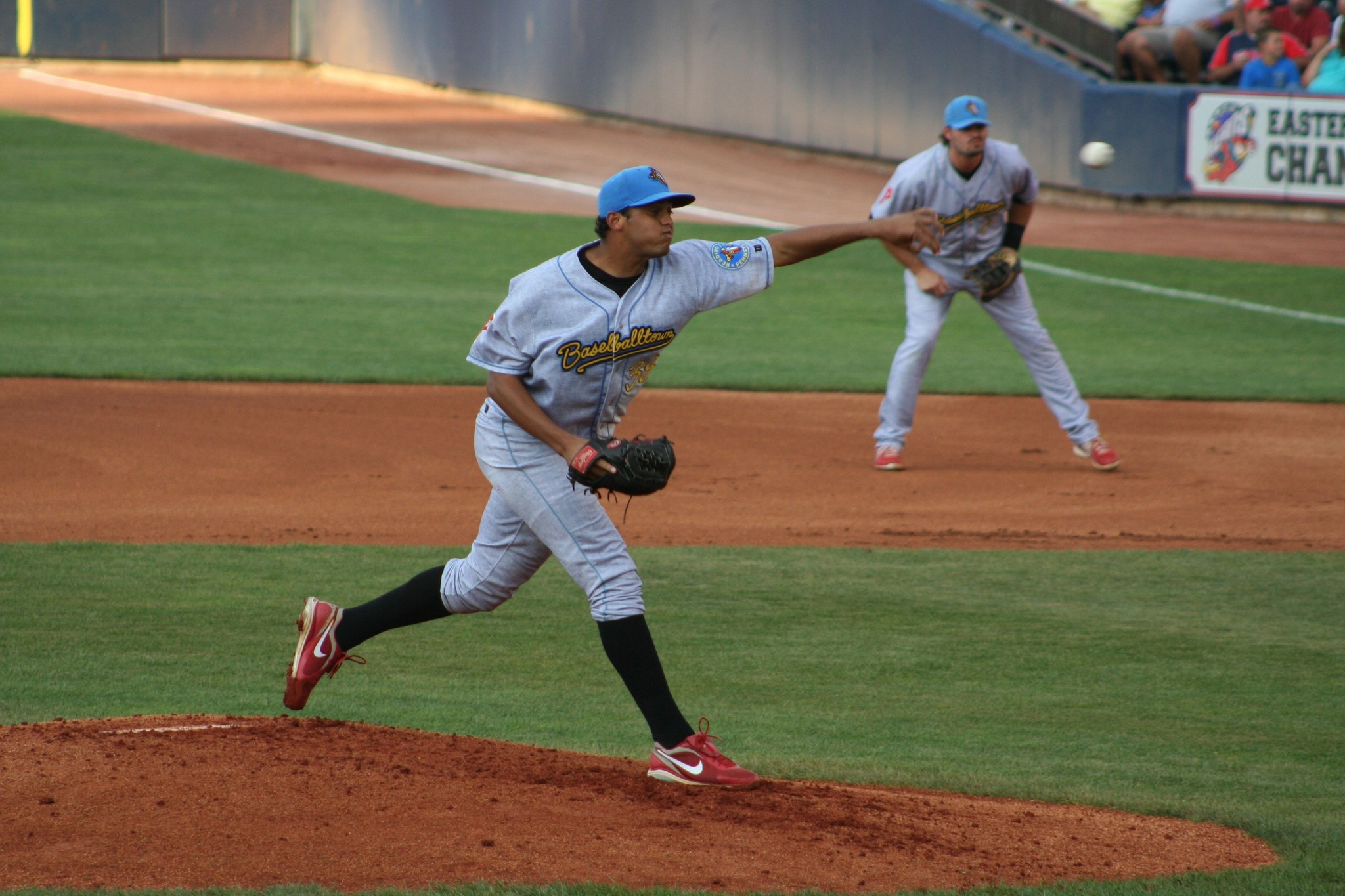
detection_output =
[597,165,695,217]
[1243,0,1272,11]
[946,94,992,128]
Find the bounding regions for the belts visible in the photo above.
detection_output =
[484,404,489,412]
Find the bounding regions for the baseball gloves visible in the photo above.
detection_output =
[566,434,675,495]
[962,244,1020,304]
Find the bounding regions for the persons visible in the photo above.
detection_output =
[868,95,1122,471]
[1057,0,1345,99]
[279,166,944,788]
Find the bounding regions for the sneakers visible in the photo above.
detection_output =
[285,597,367,711]
[645,716,761,790]
[875,446,904,469]
[1072,437,1121,470]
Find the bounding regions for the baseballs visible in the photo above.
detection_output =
[1078,141,1115,167]
